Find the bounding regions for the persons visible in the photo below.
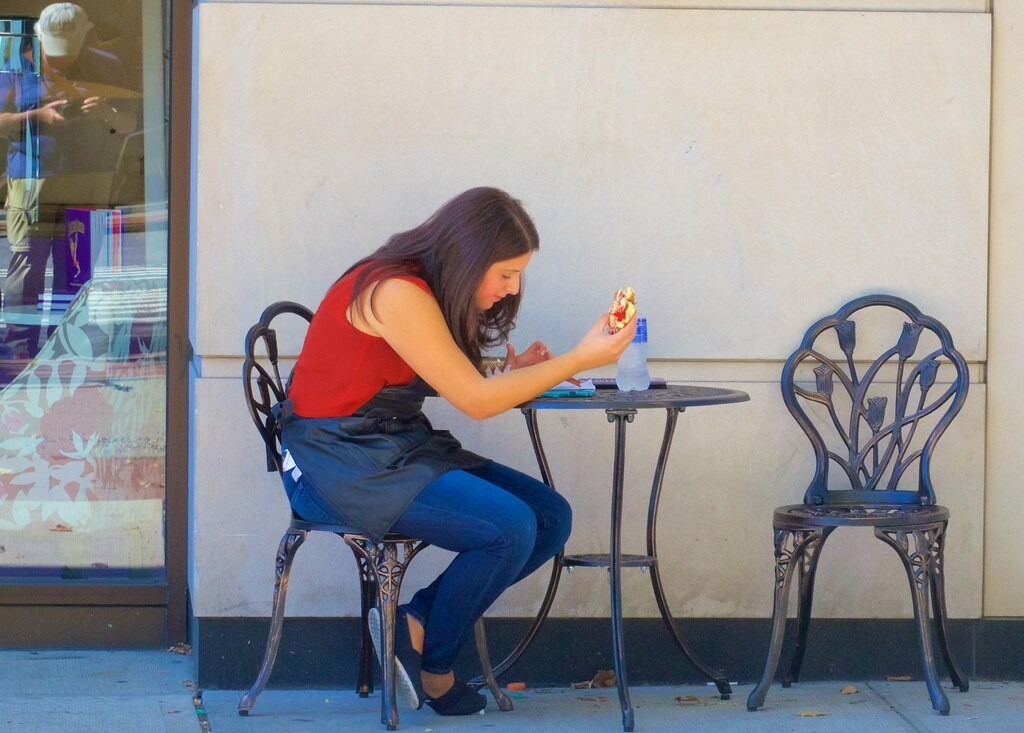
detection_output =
[0,3,140,376]
[272,185,637,716]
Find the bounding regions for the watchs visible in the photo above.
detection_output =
[103,105,119,124]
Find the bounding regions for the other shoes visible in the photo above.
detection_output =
[369,603,427,709]
[425,678,488,714]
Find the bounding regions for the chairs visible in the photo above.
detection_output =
[236,300,515,727]
[748,295,977,721]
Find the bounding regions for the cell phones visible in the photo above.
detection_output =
[538,388,597,398]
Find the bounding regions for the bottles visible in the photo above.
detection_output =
[616,310,650,391]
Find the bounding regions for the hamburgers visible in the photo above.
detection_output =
[608,287,636,333]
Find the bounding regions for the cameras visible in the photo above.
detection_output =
[54,100,85,118]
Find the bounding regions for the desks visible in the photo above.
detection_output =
[465,372,752,733]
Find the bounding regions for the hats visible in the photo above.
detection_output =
[33,3,92,58]
